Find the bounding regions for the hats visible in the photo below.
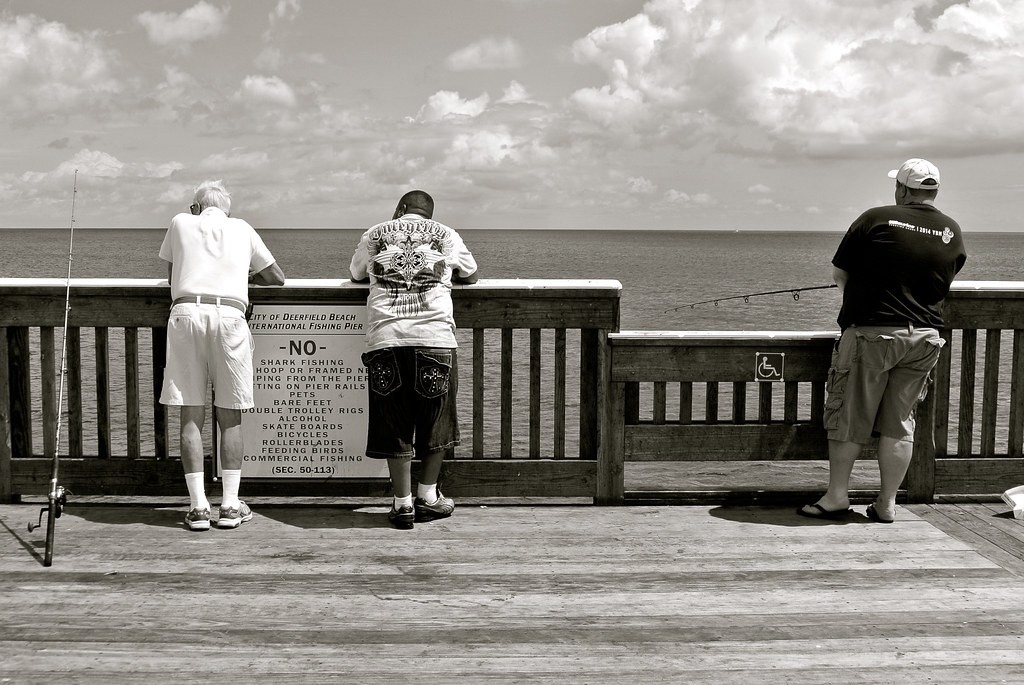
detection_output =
[888,158,942,191]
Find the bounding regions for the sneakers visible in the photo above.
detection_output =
[217,499,255,529]
[415,488,456,523]
[387,499,416,530]
[185,507,213,532]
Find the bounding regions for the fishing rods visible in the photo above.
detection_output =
[662,281,839,317]
[28,167,81,568]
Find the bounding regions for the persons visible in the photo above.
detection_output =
[350,190,479,530]
[154,181,286,529]
[798,157,968,523]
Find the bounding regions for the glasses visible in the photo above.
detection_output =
[190,203,198,214]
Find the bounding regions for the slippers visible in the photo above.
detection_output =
[866,500,899,524]
[794,502,857,525]
